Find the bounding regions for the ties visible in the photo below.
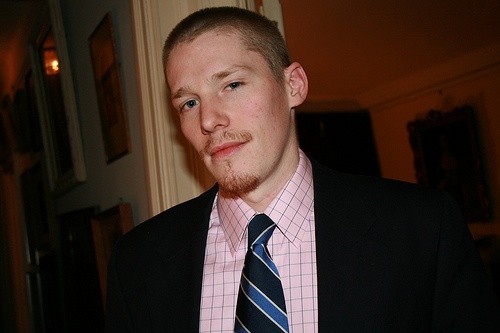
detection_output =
[233,214,290,333]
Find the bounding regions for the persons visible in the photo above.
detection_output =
[103,7,500,333]
